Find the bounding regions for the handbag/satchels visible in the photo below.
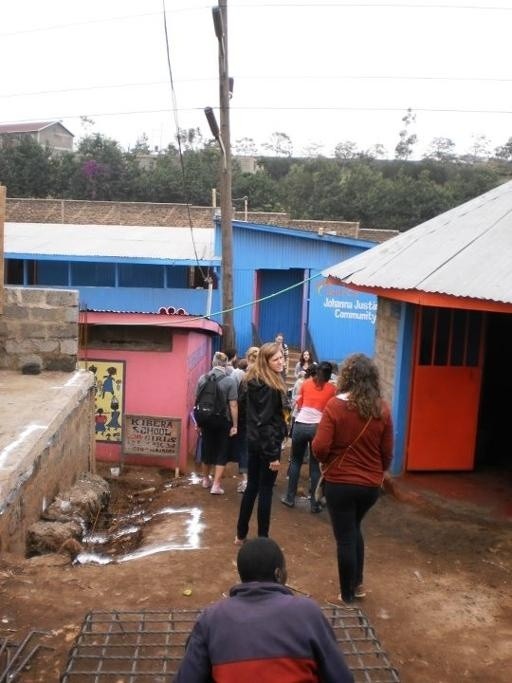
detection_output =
[314,462,325,503]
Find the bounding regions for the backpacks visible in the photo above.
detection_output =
[193,373,227,431]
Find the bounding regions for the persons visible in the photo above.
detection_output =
[192,333,338,545]
[171,536,355,683]
[312,353,394,612]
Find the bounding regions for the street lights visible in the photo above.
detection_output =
[204,0,234,357]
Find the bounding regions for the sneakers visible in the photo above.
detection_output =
[202,477,212,488]
[324,594,353,608]
[210,483,224,495]
[237,480,248,492]
[353,585,367,597]
[311,505,324,513]
[281,497,294,507]
